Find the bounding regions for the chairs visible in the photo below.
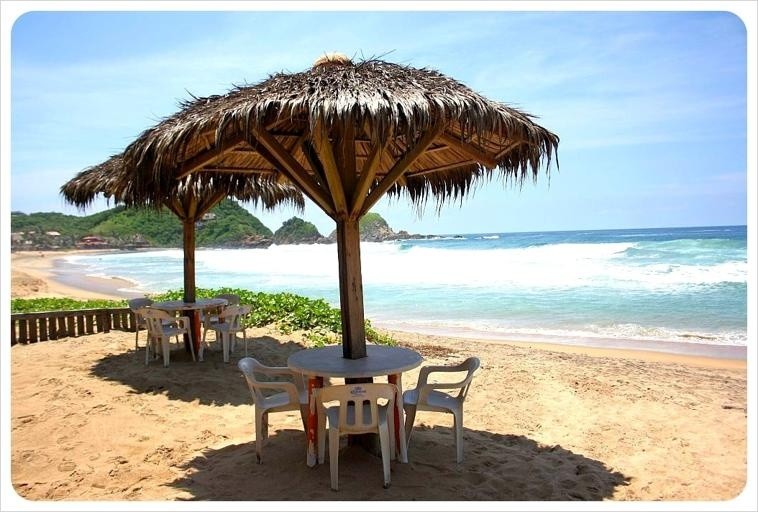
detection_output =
[236,345,481,492]
[128,294,252,367]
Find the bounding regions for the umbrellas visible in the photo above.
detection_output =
[61,153,304,361]
[114,52,560,447]
